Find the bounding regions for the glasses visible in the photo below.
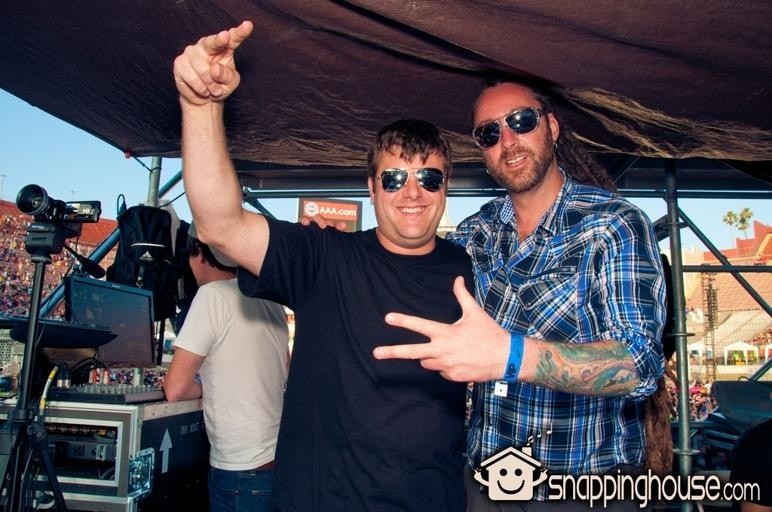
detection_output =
[377,167,447,192]
[473,107,548,151]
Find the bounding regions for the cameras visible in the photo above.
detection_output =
[16,184,102,264]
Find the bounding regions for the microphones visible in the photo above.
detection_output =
[63,244,105,278]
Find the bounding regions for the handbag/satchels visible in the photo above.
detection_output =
[106,207,180,321]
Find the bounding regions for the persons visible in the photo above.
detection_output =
[665,380,717,422]
[729,416,772,512]
[163,222,290,512]
[172,19,475,512]
[299,78,668,512]
[0,214,166,390]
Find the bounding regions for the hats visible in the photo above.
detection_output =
[188,221,239,267]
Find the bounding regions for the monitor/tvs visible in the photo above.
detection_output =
[65,275,156,368]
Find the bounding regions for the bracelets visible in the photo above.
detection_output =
[502,332,523,385]
